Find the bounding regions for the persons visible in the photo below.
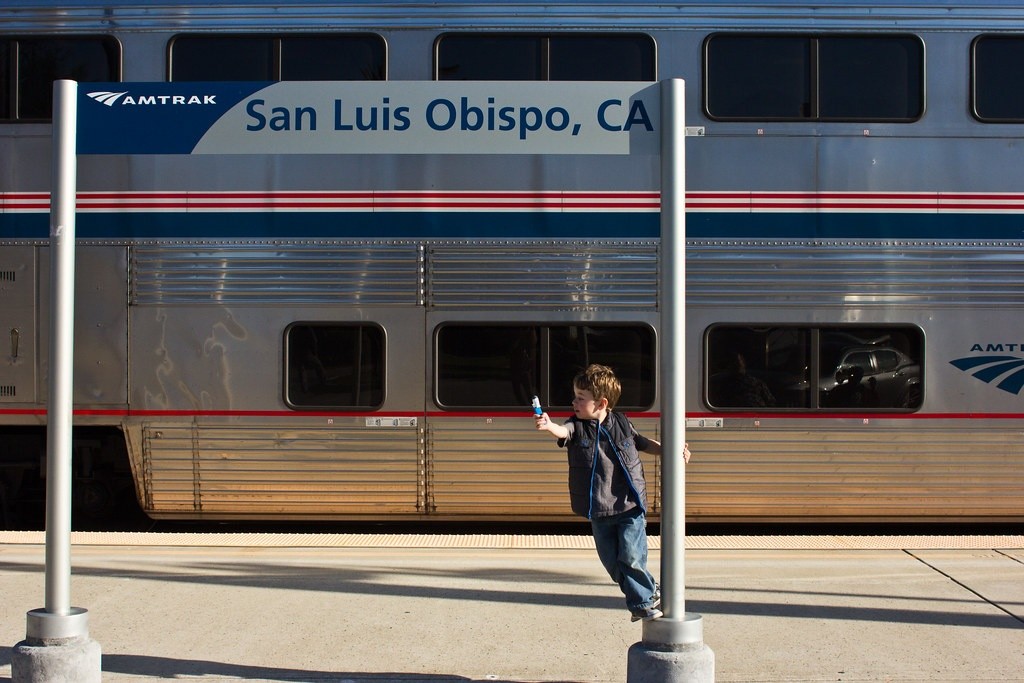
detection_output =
[534,365,691,623]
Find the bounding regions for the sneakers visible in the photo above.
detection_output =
[631,583,661,622]
[638,609,663,621]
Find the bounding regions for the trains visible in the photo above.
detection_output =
[0,2,1023,535]
